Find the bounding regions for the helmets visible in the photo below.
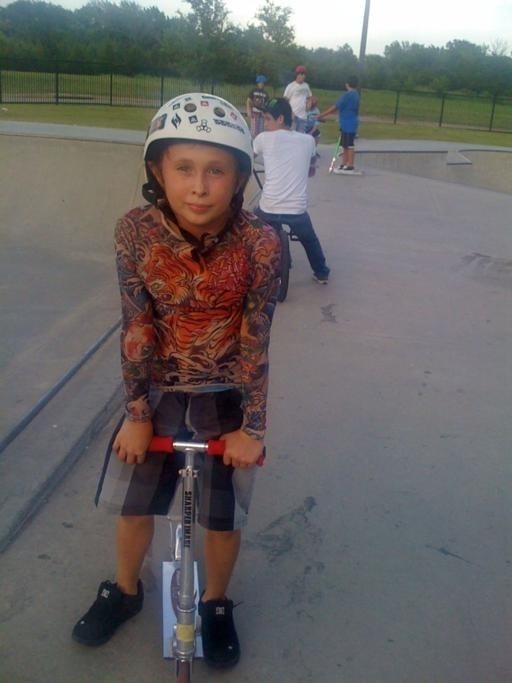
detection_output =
[295,66,305,74]
[139,90,254,210]
[255,75,267,83]
[305,96,318,112]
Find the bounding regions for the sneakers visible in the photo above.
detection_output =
[336,163,354,171]
[310,266,330,285]
[71,578,144,646]
[198,588,241,669]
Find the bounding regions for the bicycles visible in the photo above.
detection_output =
[247,159,305,301]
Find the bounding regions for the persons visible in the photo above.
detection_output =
[283,66,312,133]
[72,94,281,668]
[246,75,269,137]
[306,97,321,158]
[314,74,360,173]
[245,99,329,288]
